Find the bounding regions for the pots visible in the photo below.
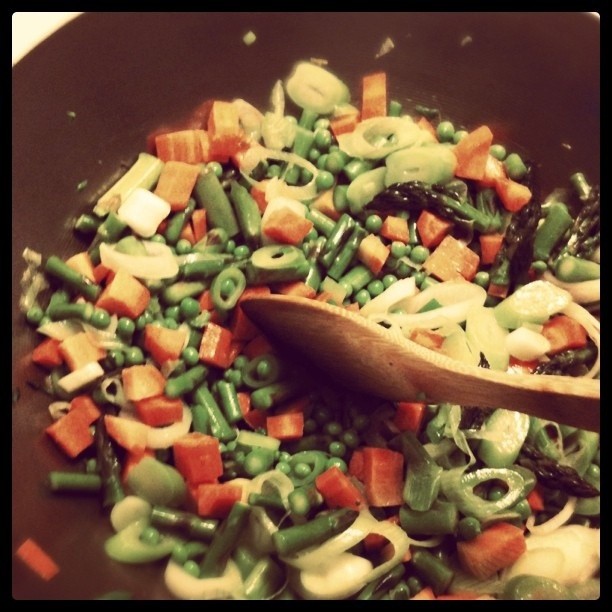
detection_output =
[12,13,601,600]
